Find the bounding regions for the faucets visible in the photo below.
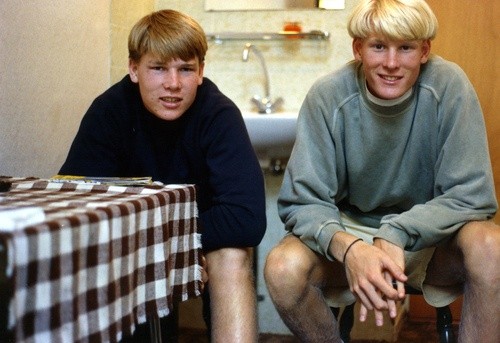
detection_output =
[241,42,283,110]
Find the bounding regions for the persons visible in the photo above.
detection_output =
[264,0,500,343]
[56,9,267,343]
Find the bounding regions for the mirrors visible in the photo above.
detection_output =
[205,0,345,12]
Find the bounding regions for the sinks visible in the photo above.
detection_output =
[242,114,300,153]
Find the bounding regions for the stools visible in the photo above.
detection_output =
[328,283,458,343]
[169,246,258,343]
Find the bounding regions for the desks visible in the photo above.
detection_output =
[0,175,203,343]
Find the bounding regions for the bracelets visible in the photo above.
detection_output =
[343,238,363,266]
